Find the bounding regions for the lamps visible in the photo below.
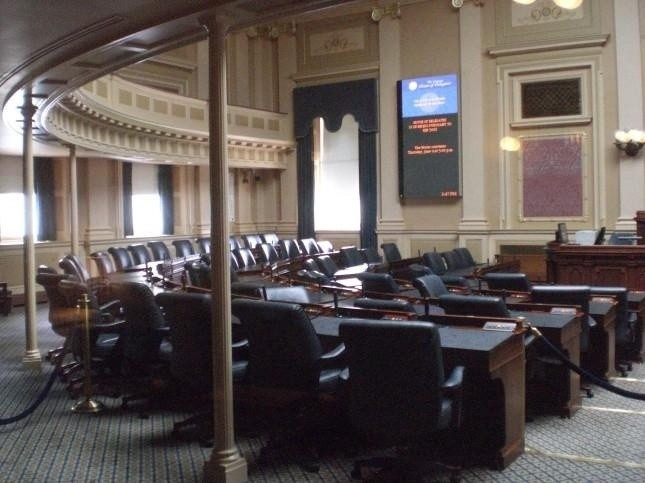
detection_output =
[612,129,645,156]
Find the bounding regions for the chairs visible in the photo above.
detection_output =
[35,229,645,483]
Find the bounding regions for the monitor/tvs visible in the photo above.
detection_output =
[556,222,569,242]
